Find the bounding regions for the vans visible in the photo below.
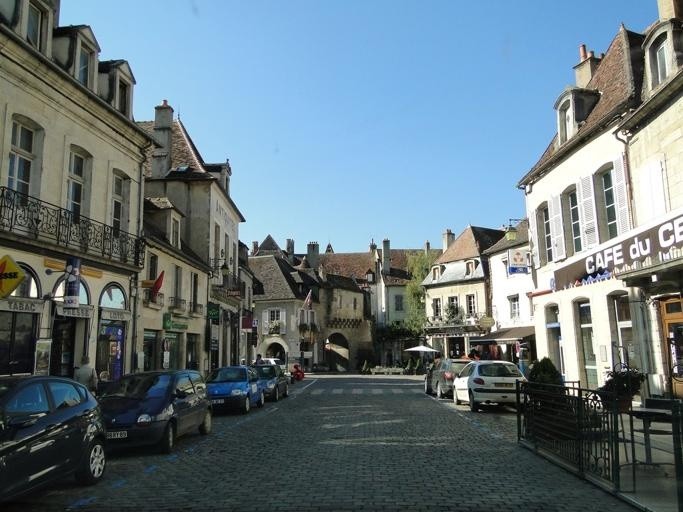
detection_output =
[252,357,286,369]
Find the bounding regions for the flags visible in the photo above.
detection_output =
[150,271,164,301]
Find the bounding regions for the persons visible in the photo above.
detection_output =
[74,356,98,398]
[256,354,265,364]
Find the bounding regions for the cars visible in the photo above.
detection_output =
[424,358,530,410]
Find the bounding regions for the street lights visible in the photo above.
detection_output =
[206,257,230,370]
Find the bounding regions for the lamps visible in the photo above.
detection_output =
[505,218,529,242]
[365,268,374,283]
[210,256,229,276]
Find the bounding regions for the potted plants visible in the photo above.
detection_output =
[594,366,649,412]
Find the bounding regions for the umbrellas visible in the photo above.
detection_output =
[404,345,440,367]
